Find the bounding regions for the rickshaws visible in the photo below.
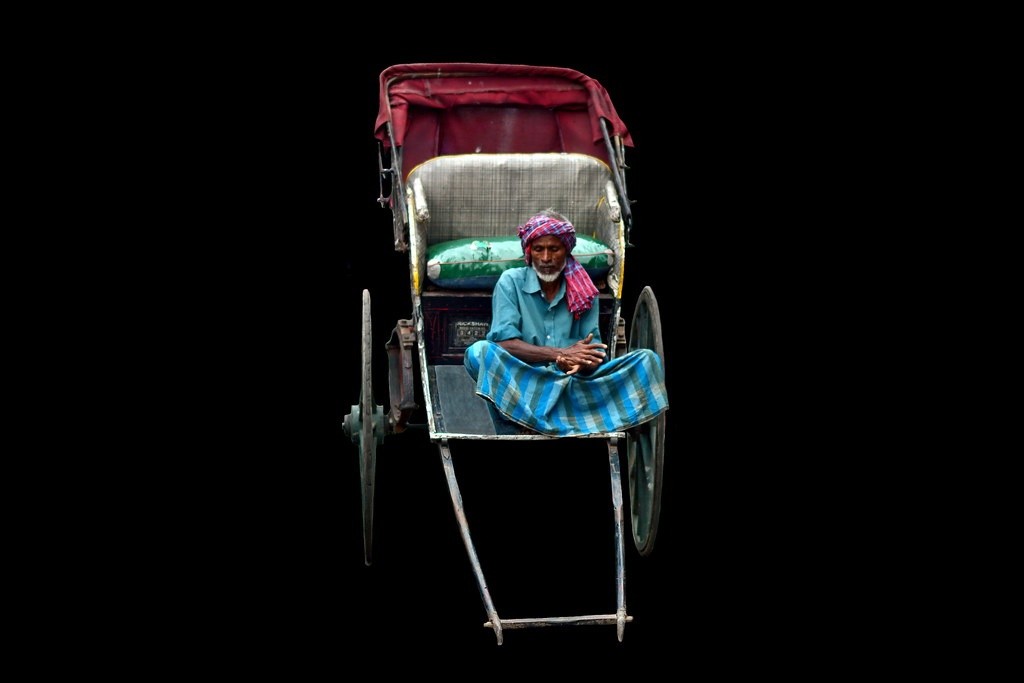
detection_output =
[342,62,671,655]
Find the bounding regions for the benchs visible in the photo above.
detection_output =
[406,152,624,300]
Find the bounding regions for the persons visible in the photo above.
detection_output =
[464,207,670,437]
